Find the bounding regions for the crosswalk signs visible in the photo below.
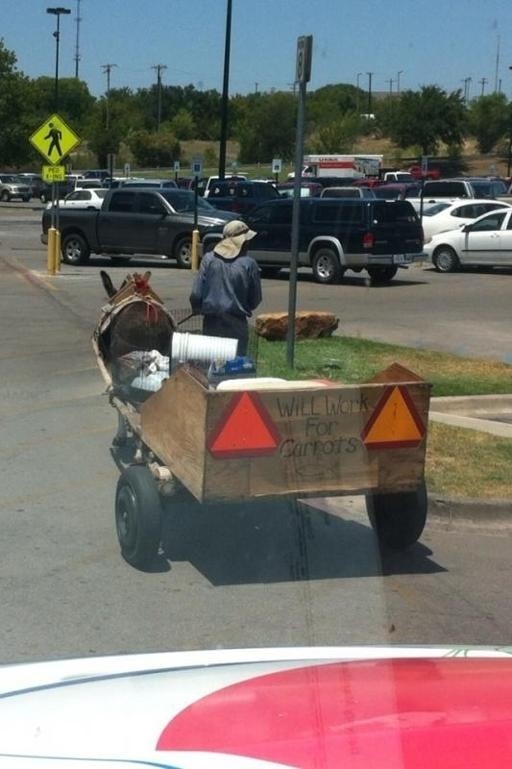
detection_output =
[28,112,82,167]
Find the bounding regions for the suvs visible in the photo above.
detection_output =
[196,197,426,283]
[171,332,239,363]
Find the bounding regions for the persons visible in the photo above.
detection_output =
[189,220,263,358]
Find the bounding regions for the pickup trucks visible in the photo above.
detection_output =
[40,187,247,269]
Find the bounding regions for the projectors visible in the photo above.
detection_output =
[91,270,434,566]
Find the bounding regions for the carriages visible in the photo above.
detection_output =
[91,270,434,566]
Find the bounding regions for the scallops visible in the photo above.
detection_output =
[97,270,175,463]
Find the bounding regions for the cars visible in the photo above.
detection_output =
[0,154,512,215]
[420,207,512,274]
[415,197,511,244]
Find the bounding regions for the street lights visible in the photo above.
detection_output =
[46,8,71,113]
[366,72,374,121]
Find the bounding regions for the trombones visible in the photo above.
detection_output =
[28,112,82,167]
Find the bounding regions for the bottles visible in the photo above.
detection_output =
[222,219,257,242]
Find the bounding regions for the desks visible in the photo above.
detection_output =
[366,72,374,121]
[46,8,71,113]
